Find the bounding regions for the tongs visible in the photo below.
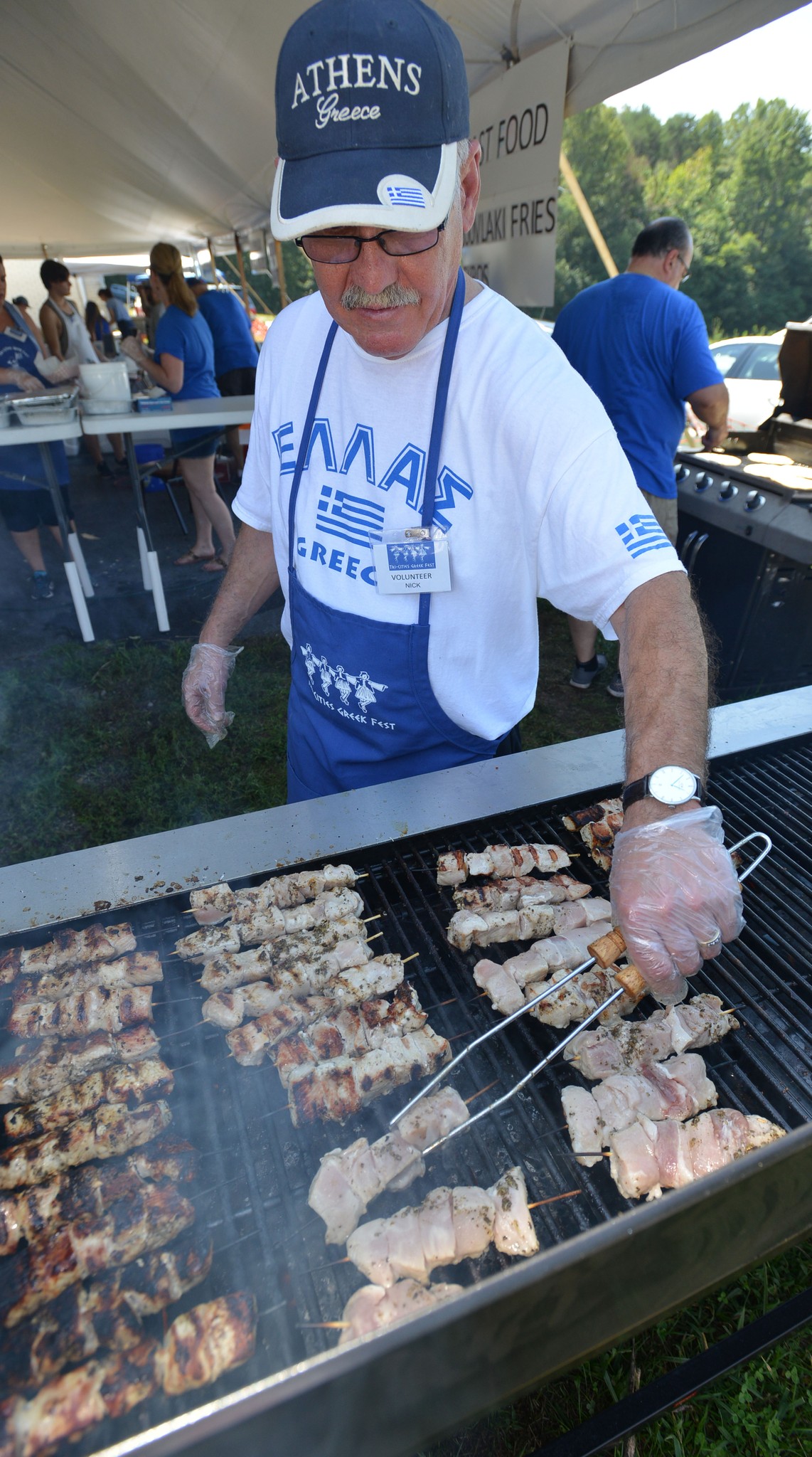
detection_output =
[386,832,772,1155]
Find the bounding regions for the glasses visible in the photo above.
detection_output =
[293,213,449,263]
[668,251,691,282]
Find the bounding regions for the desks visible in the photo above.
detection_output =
[0,394,255,643]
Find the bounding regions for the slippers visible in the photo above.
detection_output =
[202,555,228,571]
[171,549,216,566]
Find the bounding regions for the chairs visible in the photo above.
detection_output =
[752,361,779,380]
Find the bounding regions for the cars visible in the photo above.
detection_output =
[685,335,811,436]
[109,283,140,308]
[209,284,256,314]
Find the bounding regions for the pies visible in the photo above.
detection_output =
[693,452,812,490]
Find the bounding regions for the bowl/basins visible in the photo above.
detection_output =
[81,398,132,414]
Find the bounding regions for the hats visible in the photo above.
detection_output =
[16,296,30,307]
[269,0,469,242]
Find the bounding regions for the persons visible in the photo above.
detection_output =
[1,242,264,600]
[185,1,746,1008]
[548,218,738,700]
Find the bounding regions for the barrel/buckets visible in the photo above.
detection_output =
[78,361,131,400]
[134,443,166,492]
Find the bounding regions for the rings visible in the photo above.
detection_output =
[698,928,724,949]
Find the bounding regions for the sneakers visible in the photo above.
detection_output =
[570,655,608,688]
[30,571,53,600]
[606,676,625,699]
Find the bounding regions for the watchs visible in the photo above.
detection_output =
[618,766,708,814]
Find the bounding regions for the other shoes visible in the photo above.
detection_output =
[97,462,114,479]
[235,476,242,487]
[121,466,143,474]
